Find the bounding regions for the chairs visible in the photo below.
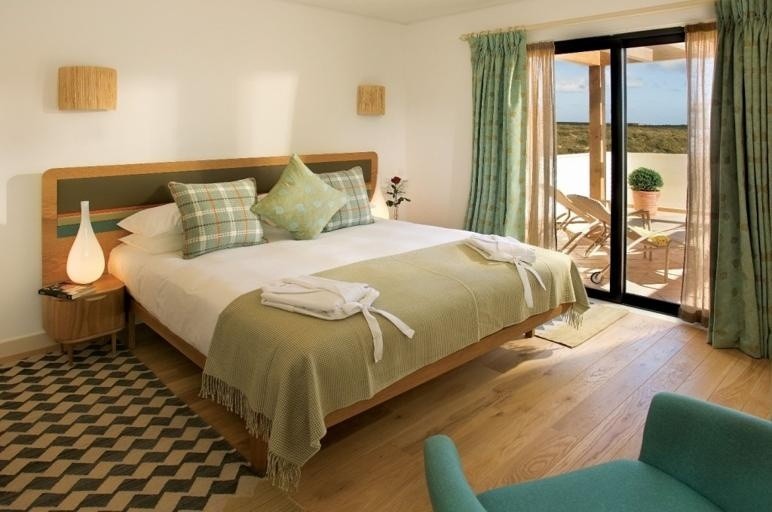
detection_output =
[548,186,607,259]
[569,194,671,285]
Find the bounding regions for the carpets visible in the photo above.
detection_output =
[0,338,297,511]
[531,302,629,349]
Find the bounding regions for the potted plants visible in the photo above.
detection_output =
[629,167,664,213]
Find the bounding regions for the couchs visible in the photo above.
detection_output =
[424,392,771,511]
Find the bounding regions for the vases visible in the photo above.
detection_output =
[393,207,401,222]
[67,199,107,286]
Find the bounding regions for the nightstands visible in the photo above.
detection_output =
[43,272,123,361]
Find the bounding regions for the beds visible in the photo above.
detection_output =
[42,153,572,449]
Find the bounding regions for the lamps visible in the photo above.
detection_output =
[357,84,387,115]
[58,65,118,112]
[369,193,388,220]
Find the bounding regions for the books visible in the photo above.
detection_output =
[38,281,97,301]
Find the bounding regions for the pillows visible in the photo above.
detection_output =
[250,154,350,240]
[306,168,373,232]
[116,232,187,257]
[170,179,267,259]
[116,197,187,240]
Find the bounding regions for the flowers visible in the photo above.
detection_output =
[386,176,412,206]
